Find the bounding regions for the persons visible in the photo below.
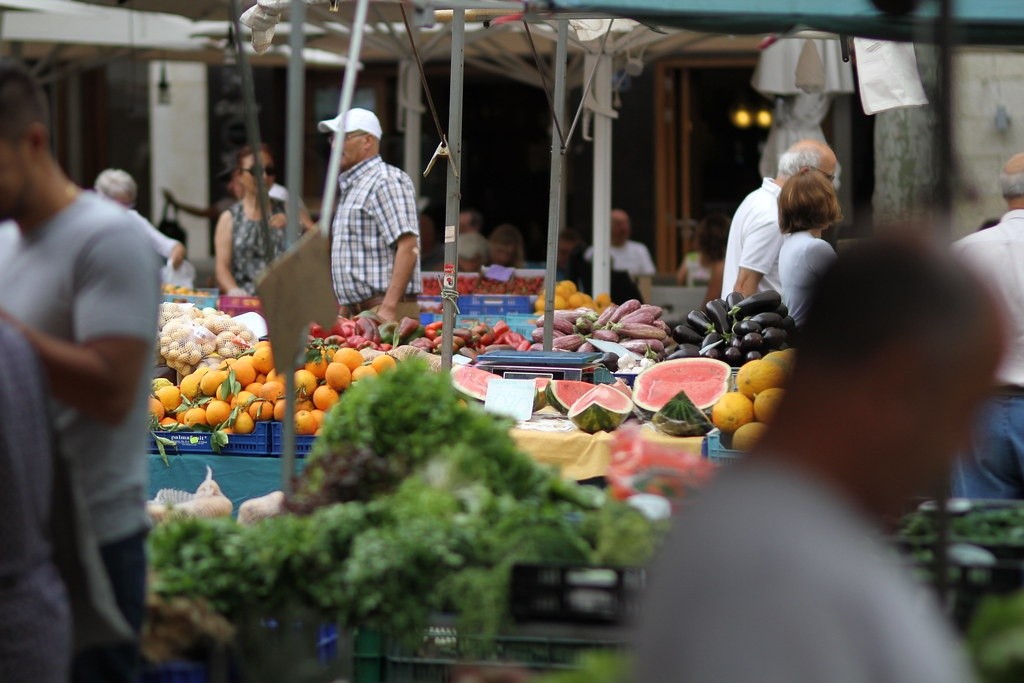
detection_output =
[720,140,836,300]
[212,142,314,297]
[0,317,78,682]
[678,213,732,285]
[459,211,482,234]
[635,227,1011,683]
[775,168,836,326]
[1,57,162,638]
[319,108,420,327]
[96,169,185,272]
[164,184,238,217]
[419,212,444,272]
[485,225,526,268]
[937,153,1024,496]
[583,207,659,307]
[456,231,489,274]
[558,229,583,267]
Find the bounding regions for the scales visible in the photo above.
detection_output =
[477,350,617,385]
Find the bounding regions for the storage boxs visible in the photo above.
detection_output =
[148,414,319,459]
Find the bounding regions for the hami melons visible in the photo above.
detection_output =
[712,348,795,454]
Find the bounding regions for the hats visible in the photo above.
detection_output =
[317,107,382,141]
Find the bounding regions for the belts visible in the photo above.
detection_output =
[351,293,418,316]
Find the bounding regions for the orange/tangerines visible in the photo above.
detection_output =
[530,274,614,318]
[150,340,397,436]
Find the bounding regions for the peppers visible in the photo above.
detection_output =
[305,309,529,362]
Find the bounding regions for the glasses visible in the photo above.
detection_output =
[240,163,280,177]
[327,133,368,144]
[809,165,835,183]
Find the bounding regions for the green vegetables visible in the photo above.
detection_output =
[138,372,655,653]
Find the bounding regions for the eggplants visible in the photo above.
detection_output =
[533,289,797,365]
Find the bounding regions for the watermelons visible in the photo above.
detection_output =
[446,355,732,435]
